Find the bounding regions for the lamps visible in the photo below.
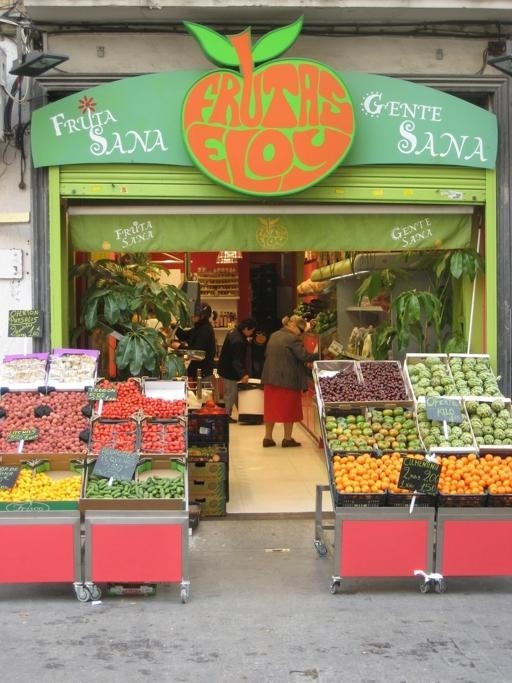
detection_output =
[487,53,512,74]
[9,52,69,76]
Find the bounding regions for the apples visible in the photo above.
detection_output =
[181,15,356,197]
[325,407,422,452]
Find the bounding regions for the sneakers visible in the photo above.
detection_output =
[282,438,300,447]
[296,325,304,333]
[229,417,237,423]
[263,439,276,447]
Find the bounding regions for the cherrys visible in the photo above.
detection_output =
[319,362,408,401]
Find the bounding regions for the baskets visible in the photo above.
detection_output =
[324,406,425,452]
[329,452,512,507]
[188,409,229,502]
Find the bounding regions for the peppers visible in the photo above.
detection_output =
[86,474,185,500]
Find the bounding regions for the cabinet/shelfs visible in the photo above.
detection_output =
[332,270,438,361]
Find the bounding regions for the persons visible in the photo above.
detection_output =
[168,303,218,393]
[217,319,256,423]
[260,315,319,448]
